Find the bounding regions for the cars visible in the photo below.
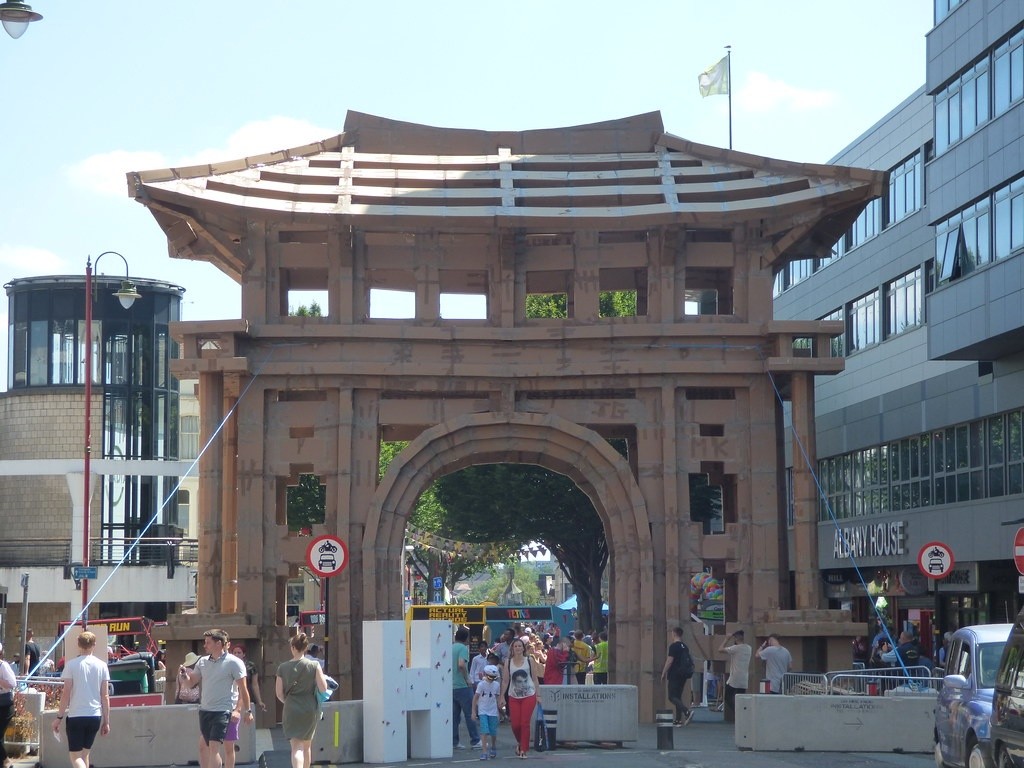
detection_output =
[932,622,1017,768]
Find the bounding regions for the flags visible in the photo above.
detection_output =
[697,55,729,97]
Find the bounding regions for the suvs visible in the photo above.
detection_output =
[990,604,1024,768]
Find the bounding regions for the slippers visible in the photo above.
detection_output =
[516,743,520,756]
[519,751,528,759]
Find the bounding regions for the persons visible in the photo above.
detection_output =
[275,633,327,768]
[0,642,17,768]
[178,629,254,768]
[155,644,265,713]
[452,629,489,751]
[471,665,506,760]
[755,633,793,695]
[852,623,954,696]
[9,629,55,681]
[470,614,608,722]
[509,670,528,698]
[498,639,542,759]
[52,631,110,768]
[661,627,695,728]
[718,629,752,713]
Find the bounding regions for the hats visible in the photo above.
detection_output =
[544,632,554,639]
[484,665,499,680]
[184,652,201,667]
[525,627,531,633]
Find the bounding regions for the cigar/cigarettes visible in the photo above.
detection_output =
[264,710,267,712]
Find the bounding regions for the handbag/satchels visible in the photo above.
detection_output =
[684,664,695,679]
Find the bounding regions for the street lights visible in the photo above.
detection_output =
[82,250,141,638]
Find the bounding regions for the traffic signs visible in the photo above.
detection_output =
[73,566,99,579]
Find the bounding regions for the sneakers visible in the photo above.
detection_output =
[472,740,489,750]
[453,743,466,749]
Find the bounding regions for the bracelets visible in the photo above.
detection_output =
[56,714,63,719]
[245,710,252,713]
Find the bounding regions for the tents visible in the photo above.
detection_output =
[557,594,609,614]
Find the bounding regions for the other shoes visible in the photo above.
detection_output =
[684,710,694,725]
[480,753,487,760]
[673,720,681,728]
[489,747,496,757]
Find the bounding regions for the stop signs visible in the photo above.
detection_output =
[1013,527,1024,575]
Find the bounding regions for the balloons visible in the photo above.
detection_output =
[691,572,724,610]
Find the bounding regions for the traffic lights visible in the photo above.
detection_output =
[688,472,714,522]
[300,610,325,626]
